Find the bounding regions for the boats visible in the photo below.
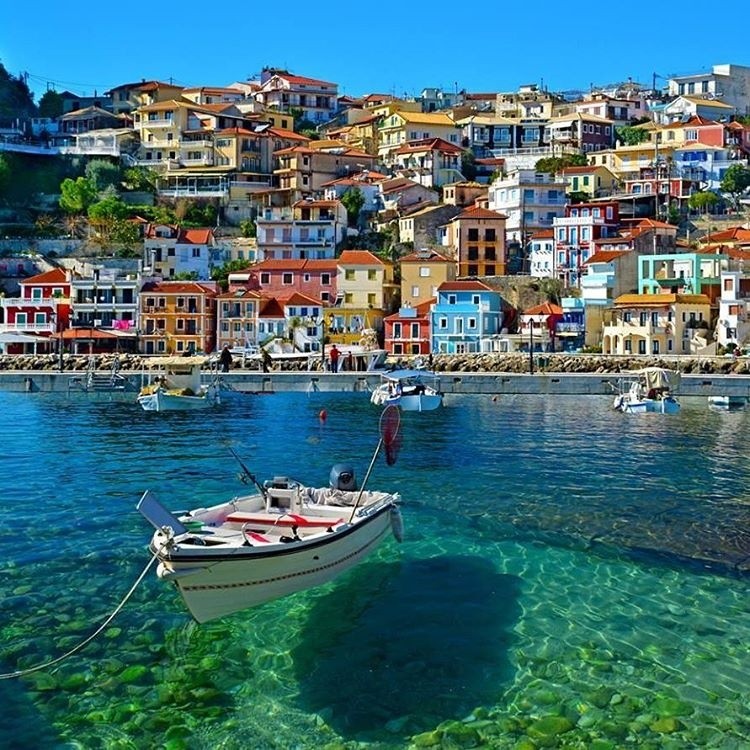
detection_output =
[363,368,445,412]
[607,366,683,414]
[135,356,223,413]
[132,443,404,626]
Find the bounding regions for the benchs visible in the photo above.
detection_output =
[226,510,343,529]
[196,524,283,545]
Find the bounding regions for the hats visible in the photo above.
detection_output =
[224,345,228,348]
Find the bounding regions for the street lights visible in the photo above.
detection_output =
[311,312,335,370]
[518,310,544,376]
[48,309,75,373]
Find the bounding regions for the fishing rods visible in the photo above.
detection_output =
[212,426,267,500]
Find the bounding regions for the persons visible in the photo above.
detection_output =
[221,345,233,372]
[330,344,341,373]
[181,347,190,357]
[262,348,271,373]
